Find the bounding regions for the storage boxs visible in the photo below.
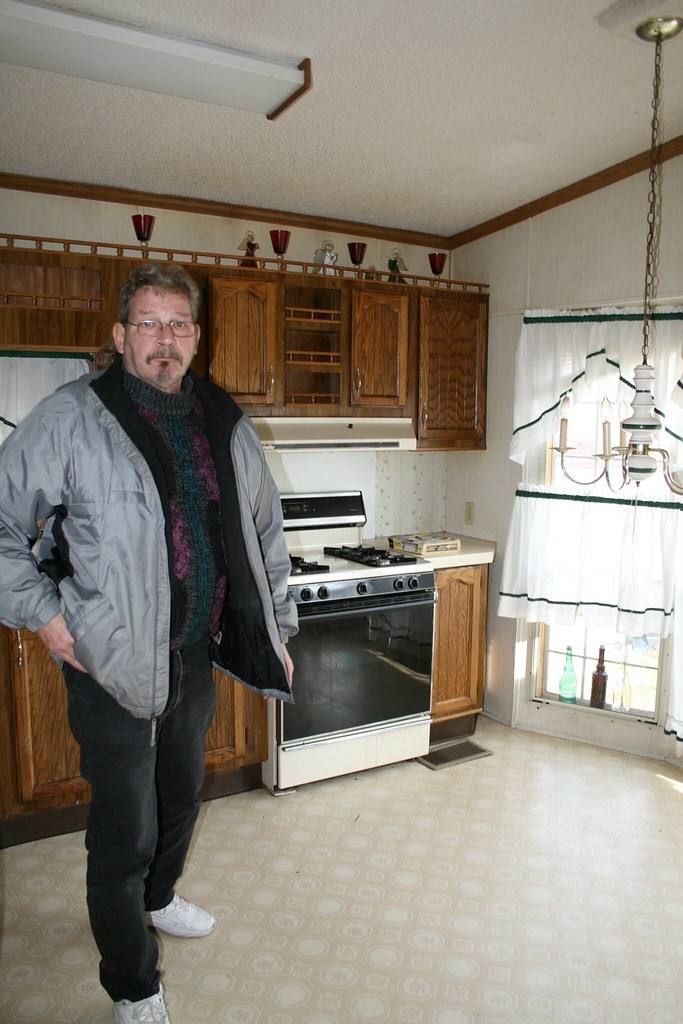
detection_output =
[388,531,461,555]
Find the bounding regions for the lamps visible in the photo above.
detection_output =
[0,0,313,121]
[550,16,683,495]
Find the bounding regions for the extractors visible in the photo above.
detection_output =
[246,417,419,453]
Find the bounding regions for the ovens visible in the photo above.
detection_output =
[255,590,437,795]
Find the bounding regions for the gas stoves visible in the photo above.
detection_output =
[259,490,434,607]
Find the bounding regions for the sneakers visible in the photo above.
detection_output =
[114,983,170,1024]
[143,895,215,937]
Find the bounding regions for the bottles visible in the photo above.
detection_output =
[590,646,608,709]
[613,655,632,714]
[559,646,576,706]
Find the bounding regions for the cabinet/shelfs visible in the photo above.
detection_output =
[429,563,490,746]
[0,232,489,451]
[1,624,269,850]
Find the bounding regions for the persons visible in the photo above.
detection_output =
[0,265,299,1024]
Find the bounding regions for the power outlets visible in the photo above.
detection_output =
[465,502,475,526]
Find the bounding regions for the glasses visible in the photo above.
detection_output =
[126,318,195,338]
